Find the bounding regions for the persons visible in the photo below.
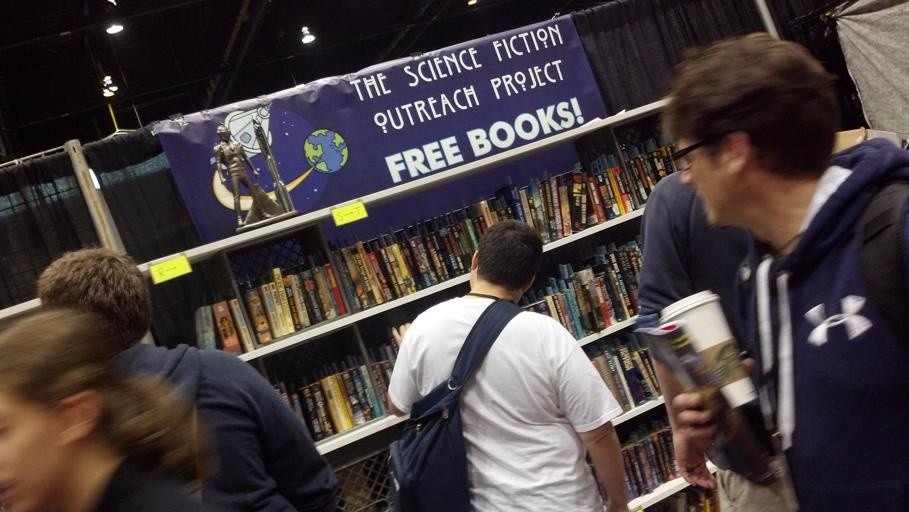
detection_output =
[633,171,792,512]
[36,248,337,512]
[0,302,223,512]
[213,123,274,227]
[660,31,909,512]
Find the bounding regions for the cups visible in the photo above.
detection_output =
[654,289,760,413]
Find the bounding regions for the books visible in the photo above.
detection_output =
[194,141,719,512]
[633,322,776,485]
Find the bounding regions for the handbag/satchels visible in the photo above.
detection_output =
[386,376,470,512]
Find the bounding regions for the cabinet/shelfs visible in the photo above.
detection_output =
[123,82,783,512]
